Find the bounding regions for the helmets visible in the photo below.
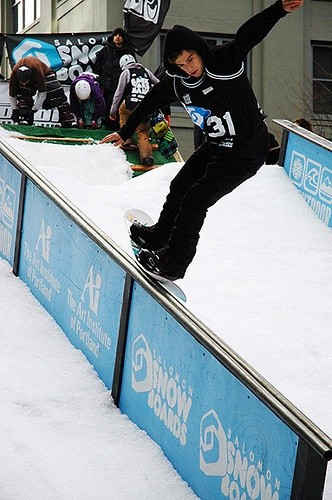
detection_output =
[75,80,92,100]
[119,54,136,71]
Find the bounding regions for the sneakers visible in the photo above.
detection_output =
[130,222,169,252]
[137,247,185,281]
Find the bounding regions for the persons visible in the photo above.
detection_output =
[95,27,138,131]
[70,73,107,129]
[8,56,77,128]
[147,58,311,167]
[101,0,303,281]
[108,54,161,166]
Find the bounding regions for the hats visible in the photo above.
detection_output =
[111,28,125,41]
[16,65,31,84]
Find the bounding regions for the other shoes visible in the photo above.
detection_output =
[143,157,154,167]
[59,113,78,128]
[17,113,34,126]
[123,144,130,147]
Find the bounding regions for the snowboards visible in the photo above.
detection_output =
[149,112,180,160]
[123,207,189,303]
